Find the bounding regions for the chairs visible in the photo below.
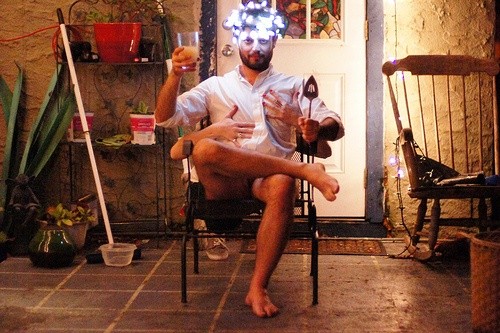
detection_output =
[382,55,500,262]
[181,114,318,305]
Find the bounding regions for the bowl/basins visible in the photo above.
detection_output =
[98,242,137,267]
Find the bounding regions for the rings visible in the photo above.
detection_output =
[239,133,243,137]
[275,100,279,105]
[232,139,237,143]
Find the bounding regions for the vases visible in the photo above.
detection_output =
[74,112,94,140]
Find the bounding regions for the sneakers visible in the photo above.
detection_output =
[202,227,229,260]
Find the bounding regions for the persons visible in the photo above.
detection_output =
[153,4,345,318]
[170,89,332,261]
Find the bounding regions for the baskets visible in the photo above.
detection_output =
[471,231,500,333]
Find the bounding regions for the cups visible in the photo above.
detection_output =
[177,31,199,72]
[138,41,157,63]
[62,40,92,63]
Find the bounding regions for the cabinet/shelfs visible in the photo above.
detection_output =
[57,0,167,249]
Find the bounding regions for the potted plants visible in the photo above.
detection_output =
[87,9,145,63]
[28,203,95,267]
[129,101,156,145]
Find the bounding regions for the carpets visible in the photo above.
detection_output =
[239,238,388,256]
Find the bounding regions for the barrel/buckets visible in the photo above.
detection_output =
[94,22,142,62]
